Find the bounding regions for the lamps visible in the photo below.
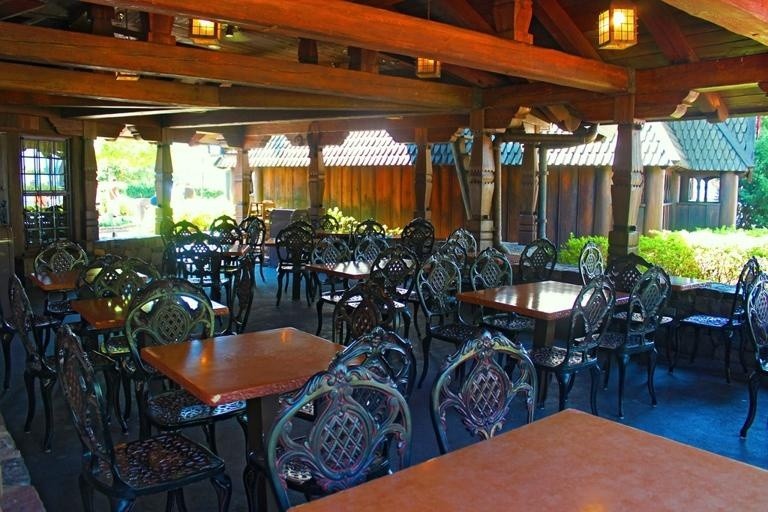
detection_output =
[415,58,440,78]
[188,18,220,46]
[114,70,139,81]
[597,4,638,51]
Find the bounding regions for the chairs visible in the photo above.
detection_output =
[231,216,266,283]
[179,233,232,327]
[311,235,363,346]
[263,363,411,511]
[0,300,62,392]
[160,216,199,278]
[278,221,315,294]
[204,223,243,305]
[126,279,251,511]
[575,266,672,420]
[161,242,177,279]
[191,255,254,341]
[10,272,130,455]
[668,259,759,384]
[350,219,385,261]
[55,324,232,511]
[471,247,535,384]
[290,209,312,228]
[529,276,618,418]
[400,218,434,289]
[242,328,416,512]
[415,259,485,389]
[353,233,402,305]
[738,271,768,439]
[342,246,419,347]
[429,331,536,453]
[604,252,674,374]
[278,281,396,422]
[175,220,201,243]
[517,239,558,284]
[315,213,340,232]
[579,240,604,287]
[398,240,467,340]
[92,254,161,422]
[33,240,89,354]
[122,278,212,442]
[275,224,315,309]
[417,252,499,391]
[75,254,123,297]
[209,215,238,231]
